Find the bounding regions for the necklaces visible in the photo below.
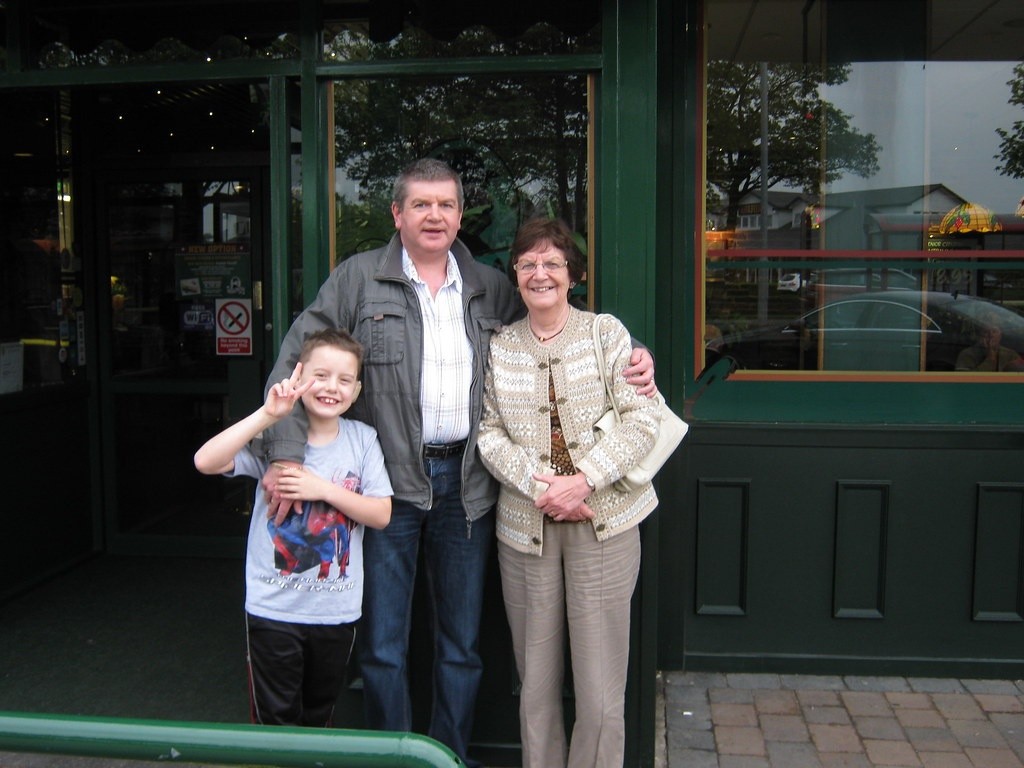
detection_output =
[530,307,570,342]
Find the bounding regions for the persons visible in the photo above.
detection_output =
[955,311,1024,371]
[474,218,660,768]
[260,156,657,768]
[194,328,394,728]
[705,325,724,369]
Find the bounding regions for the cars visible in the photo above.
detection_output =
[776,272,818,292]
[806,268,920,307]
[705,291,1024,373]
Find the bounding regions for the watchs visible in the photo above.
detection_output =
[586,475,595,491]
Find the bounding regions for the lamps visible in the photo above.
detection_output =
[939,202,1003,235]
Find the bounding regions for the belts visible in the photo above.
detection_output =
[423,442,466,459]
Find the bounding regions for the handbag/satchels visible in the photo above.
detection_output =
[593,379,690,492]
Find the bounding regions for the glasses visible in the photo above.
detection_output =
[513,259,568,274]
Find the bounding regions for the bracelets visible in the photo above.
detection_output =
[271,462,300,471]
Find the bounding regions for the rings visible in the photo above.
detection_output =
[551,511,556,516]
[651,379,655,383]
